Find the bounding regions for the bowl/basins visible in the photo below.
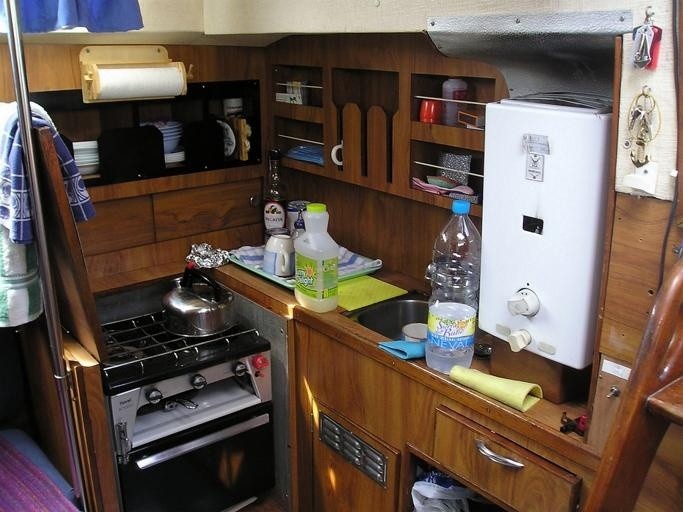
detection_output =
[72,141,100,175]
[140,120,184,153]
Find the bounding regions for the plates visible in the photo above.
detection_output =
[165,152,186,163]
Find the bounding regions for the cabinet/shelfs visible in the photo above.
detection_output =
[309,397,399,511]
[71,176,260,257]
[270,64,325,168]
[407,72,496,207]
[584,356,682,511]
[430,407,583,512]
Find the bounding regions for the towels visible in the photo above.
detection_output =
[227,243,383,286]
[0,99,96,328]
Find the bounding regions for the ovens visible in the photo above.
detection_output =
[119,401,277,512]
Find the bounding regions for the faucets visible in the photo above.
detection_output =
[424,256,461,282]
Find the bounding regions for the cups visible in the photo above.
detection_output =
[401,322,428,342]
[419,100,442,125]
[264,229,294,277]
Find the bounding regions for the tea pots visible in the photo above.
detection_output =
[162,261,236,337]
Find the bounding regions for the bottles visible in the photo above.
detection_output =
[442,76,468,127]
[425,197,482,374]
[263,146,287,229]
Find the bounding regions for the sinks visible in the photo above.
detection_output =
[342,288,434,341]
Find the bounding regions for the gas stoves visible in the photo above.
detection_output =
[102,308,273,394]
